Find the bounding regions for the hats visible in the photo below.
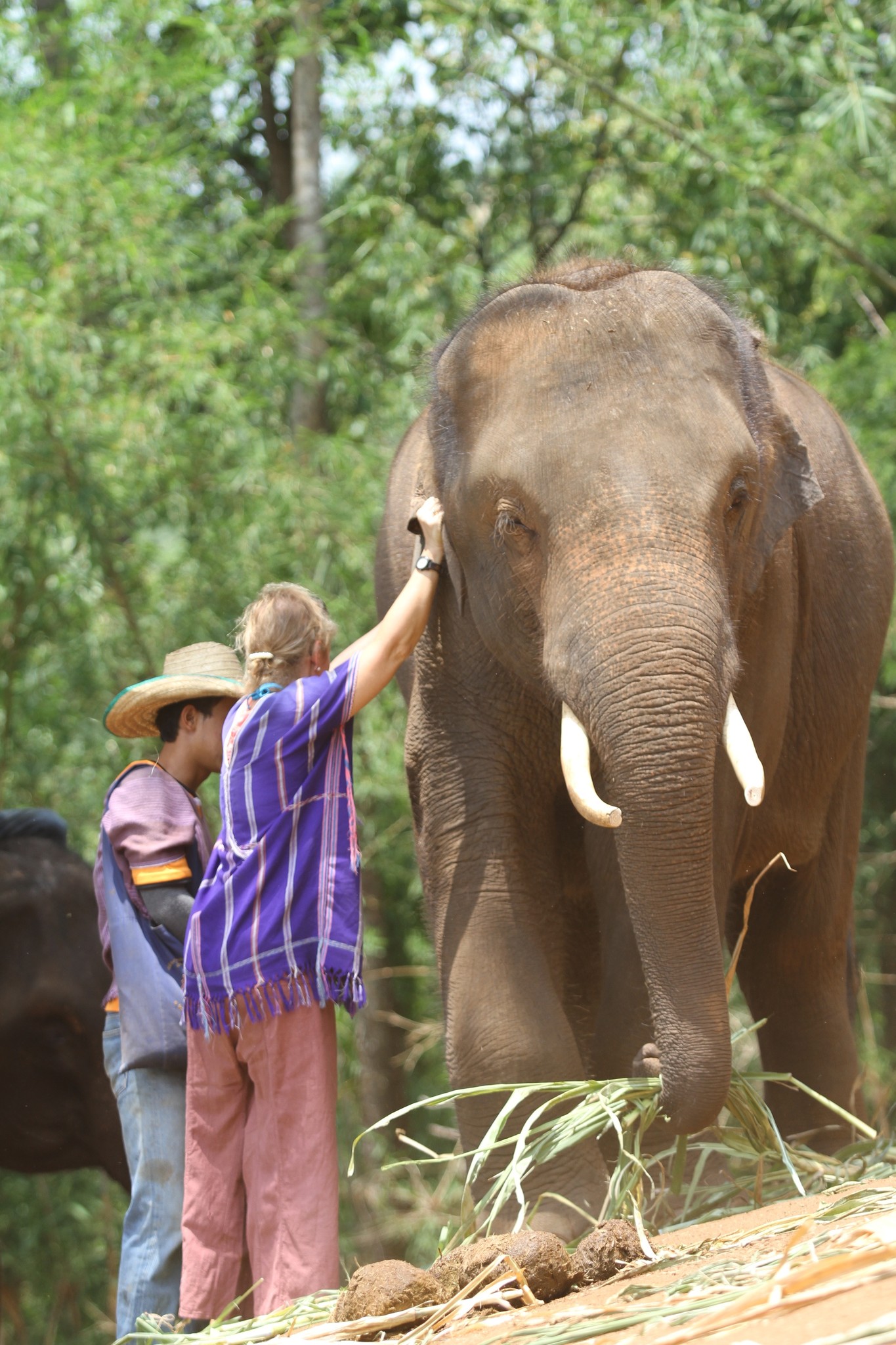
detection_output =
[103,641,244,739]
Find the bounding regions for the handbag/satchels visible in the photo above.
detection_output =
[100,759,203,1074]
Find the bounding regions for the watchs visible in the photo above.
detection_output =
[414,557,445,577]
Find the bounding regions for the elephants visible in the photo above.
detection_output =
[0,809,131,1195]
[372,242,896,1268]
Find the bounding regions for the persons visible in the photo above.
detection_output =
[176,496,446,1331]
[95,640,247,1345]
